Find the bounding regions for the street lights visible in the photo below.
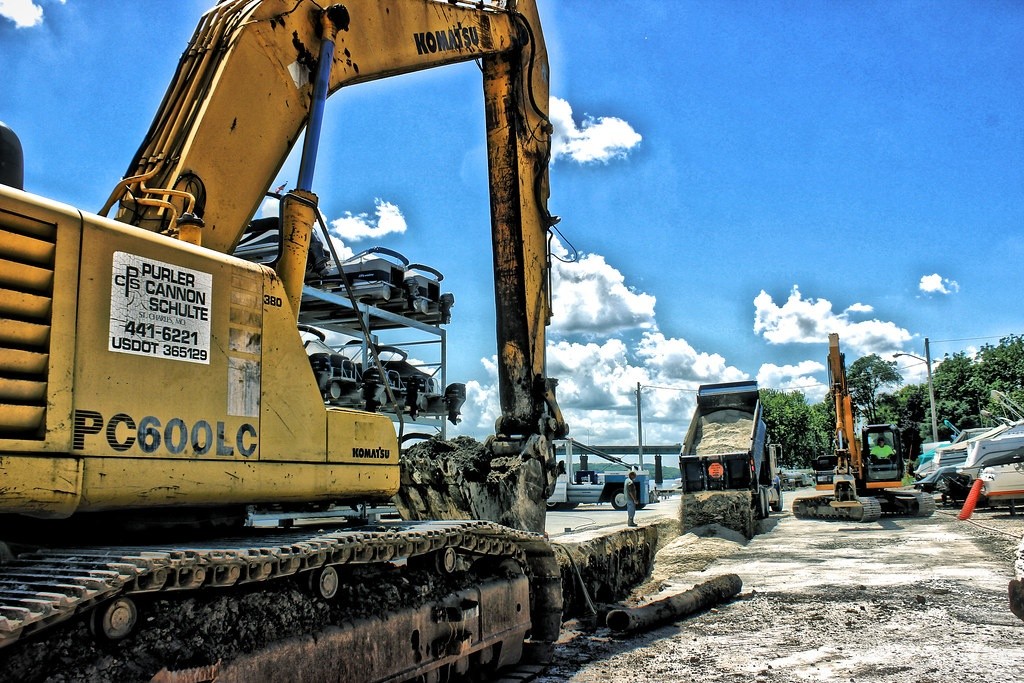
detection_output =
[892,338,938,442]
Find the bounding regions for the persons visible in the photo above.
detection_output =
[624,472,638,526]
[870,437,894,459]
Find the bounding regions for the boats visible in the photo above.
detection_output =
[913,418,1024,505]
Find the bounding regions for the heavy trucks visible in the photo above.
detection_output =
[678,380,783,519]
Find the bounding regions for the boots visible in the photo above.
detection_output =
[628,516,638,527]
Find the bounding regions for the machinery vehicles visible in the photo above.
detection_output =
[546,438,660,511]
[0,0,578,682]
[792,333,936,524]
